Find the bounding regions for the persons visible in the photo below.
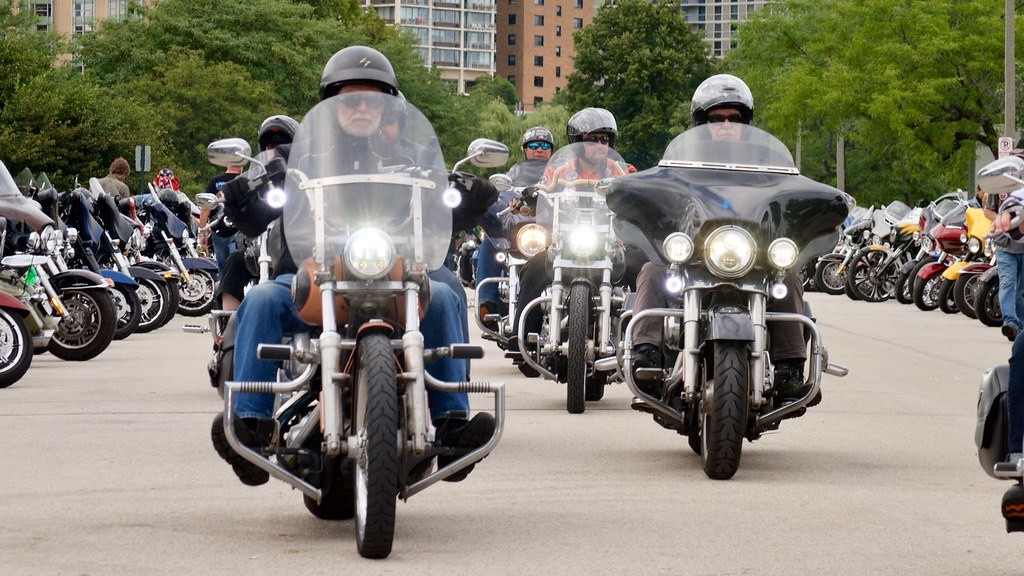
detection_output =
[200,46,495,486]
[89,157,131,199]
[967,139,1024,467]
[631,74,822,407]
[476,108,651,352]
[132,189,178,226]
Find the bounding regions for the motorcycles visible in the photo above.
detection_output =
[596,120,855,482]
[36,167,245,341]
[207,90,508,559]
[0,161,57,389]
[7,167,120,360]
[452,158,561,359]
[800,153,1024,534]
[488,140,647,414]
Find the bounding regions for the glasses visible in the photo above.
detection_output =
[707,114,744,124]
[583,136,611,145]
[333,95,389,109]
[261,136,294,150]
[525,142,552,150]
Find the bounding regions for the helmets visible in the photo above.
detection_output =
[258,114,309,155]
[319,46,399,110]
[522,127,554,154]
[566,106,618,145]
[690,74,754,128]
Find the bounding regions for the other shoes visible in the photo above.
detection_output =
[631,348,664,401]
[1002,323,1019,342]
[212,412,270,486]
[773,366,821,409]
[478,302,500,334]
[507,335,531,368]
[1003,483,1024,534]
[436,412,495,481]
